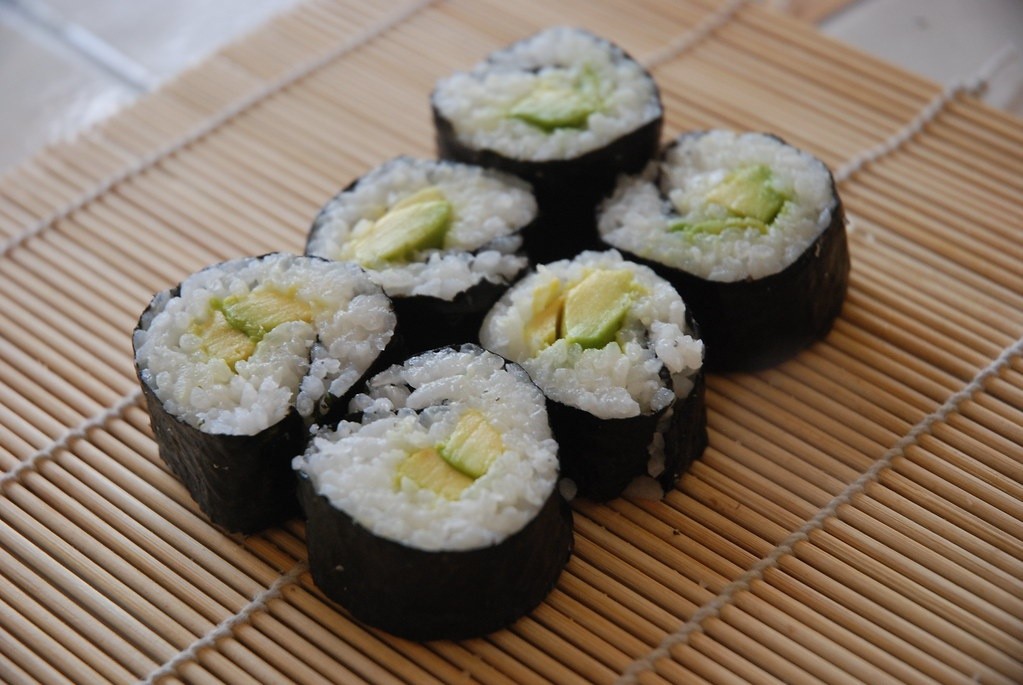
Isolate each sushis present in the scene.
[126,27,852,643]
